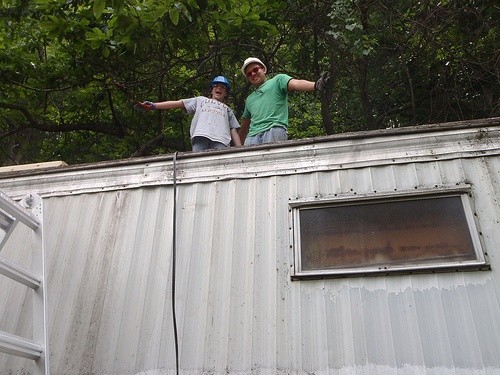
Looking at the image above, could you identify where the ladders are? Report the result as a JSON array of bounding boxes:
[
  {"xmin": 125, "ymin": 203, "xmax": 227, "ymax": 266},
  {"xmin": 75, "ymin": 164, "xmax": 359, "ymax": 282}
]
[{"xmin": 0, "ymin": 188, "xmax": 50, "ymax": 375}]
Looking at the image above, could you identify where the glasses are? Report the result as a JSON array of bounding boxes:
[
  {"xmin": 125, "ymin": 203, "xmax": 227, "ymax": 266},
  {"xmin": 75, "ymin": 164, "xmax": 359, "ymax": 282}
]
[
  {"xmin": 246, "ymin": 67, "xmax": 263, "ymax": 77},
  {"xmin": 212, "ymin": 85, "xmax": 227, "ymax": 89}
]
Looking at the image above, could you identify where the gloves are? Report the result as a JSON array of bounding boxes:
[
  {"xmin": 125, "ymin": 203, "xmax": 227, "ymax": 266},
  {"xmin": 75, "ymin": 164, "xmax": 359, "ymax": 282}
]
[
  {"xmin": 314, "ymin": 71, "xmax": 331, "ymax": 94},
  {"xmin": 139, "ymin": 101, "xmax": 155, "ymax": 110}
]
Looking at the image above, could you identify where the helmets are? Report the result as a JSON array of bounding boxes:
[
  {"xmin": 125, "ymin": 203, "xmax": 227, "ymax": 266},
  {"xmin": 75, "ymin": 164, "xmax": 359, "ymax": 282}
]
[{"xmin": 210, "ymin": 76, "xmax": 230, "ymax": 92}]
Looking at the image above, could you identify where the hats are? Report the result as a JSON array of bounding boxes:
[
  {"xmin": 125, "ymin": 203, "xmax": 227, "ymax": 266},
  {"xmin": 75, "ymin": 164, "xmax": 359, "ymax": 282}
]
[{"xmin": 241, "ymin": 57, "xmax": 267, "ymax": 78}]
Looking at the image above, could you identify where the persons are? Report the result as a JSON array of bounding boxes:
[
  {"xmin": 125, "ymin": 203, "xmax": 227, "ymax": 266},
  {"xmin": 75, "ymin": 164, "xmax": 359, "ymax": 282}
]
[
  {"xmin": 138, "ymin": 76, "xmax": 242, "ymax": 152},
  {"xmin": 240, "ymin": 57, "xmax": 330, "ymax": 145}
]
